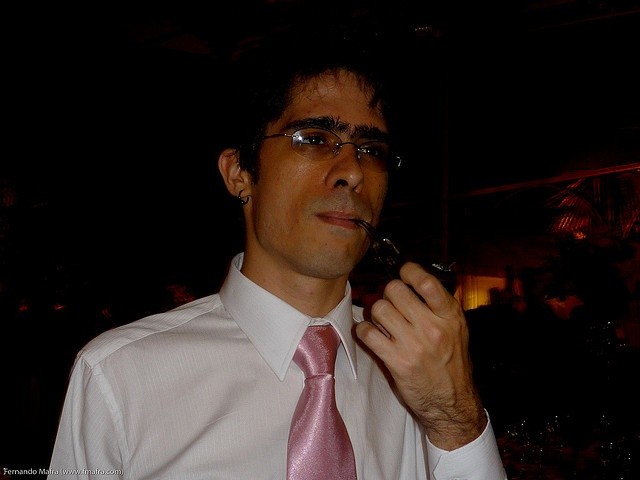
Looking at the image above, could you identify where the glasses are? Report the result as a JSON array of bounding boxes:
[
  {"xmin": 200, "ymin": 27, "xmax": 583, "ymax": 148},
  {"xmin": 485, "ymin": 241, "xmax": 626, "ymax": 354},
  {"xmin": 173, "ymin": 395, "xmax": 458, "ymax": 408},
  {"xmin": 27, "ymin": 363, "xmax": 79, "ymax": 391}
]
[{"xmin": 261, "ymin": 126, "xmax": 393, "ymax": 169}]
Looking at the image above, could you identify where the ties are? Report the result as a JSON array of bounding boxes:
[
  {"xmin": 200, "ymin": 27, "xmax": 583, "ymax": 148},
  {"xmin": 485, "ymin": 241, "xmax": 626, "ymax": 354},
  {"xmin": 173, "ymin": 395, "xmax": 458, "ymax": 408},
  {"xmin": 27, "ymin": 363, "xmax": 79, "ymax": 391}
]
[{"xmin": 288, "ymin": 327, "xmax": 357, "ymax": 480}]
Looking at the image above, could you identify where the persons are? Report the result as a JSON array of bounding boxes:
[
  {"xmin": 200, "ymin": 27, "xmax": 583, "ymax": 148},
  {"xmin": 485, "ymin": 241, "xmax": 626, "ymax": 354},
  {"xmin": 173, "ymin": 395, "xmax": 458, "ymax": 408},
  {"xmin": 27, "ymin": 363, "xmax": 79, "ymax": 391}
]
[{"xmin": 47, "ymin": 39, "xmax": 509, "ymax": 480}]
